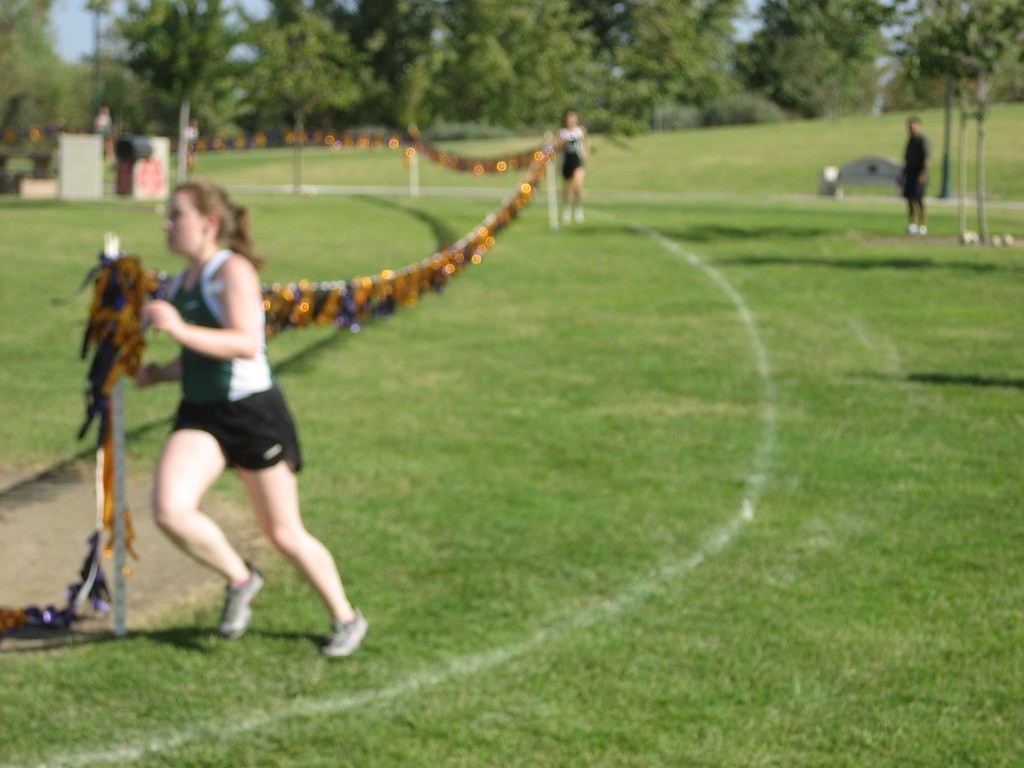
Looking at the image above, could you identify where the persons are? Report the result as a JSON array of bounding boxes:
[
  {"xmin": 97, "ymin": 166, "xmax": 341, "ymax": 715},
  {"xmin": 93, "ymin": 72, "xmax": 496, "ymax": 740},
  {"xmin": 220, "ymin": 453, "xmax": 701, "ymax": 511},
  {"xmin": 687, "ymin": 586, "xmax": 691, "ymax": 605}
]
[
  {"xmin": 186, "ymin": 119, "xmax": 201, "ymax": 171},
  {"xmin": 93, "ymin": 105, "xmax": 112, "ymax": 152},
  {"xmin": 138, "ymin": 178, "xmax": 365, "ymax": 657},
  {"xmin": 897, "ymin": 117, "xmax": 932, "ymax": 235},
  {"xmin": 556, "ymin": 110, "xmax": 591, "ymax": 226}
]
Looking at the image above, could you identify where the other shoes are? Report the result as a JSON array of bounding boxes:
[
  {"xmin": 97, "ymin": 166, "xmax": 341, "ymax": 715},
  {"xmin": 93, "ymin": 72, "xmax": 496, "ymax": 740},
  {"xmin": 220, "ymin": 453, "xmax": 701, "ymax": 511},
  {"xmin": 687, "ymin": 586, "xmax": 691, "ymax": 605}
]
[
  {"xmin": 908, "ymin": 223, "xmax": 927, "ymax": 235},
  {"xmin": 562, "ymin": 208, "xmax": 572, "ymax": 226},
  {"xmin": 574, "ymin": 206, "xmax": 584, "ymax": 223}
]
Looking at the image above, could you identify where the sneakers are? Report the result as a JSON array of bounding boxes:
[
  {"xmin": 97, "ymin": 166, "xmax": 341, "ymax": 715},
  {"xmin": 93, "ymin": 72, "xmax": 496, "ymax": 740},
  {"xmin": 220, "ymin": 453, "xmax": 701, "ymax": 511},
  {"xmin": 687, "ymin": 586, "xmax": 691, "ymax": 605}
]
[
  {"xmin": 219, "ymin": 561, "xmax": 265, "ymax": 640},
  {"xmin": 320, "ymin": 606, "xmax": 369, "ymax": 656}
]
